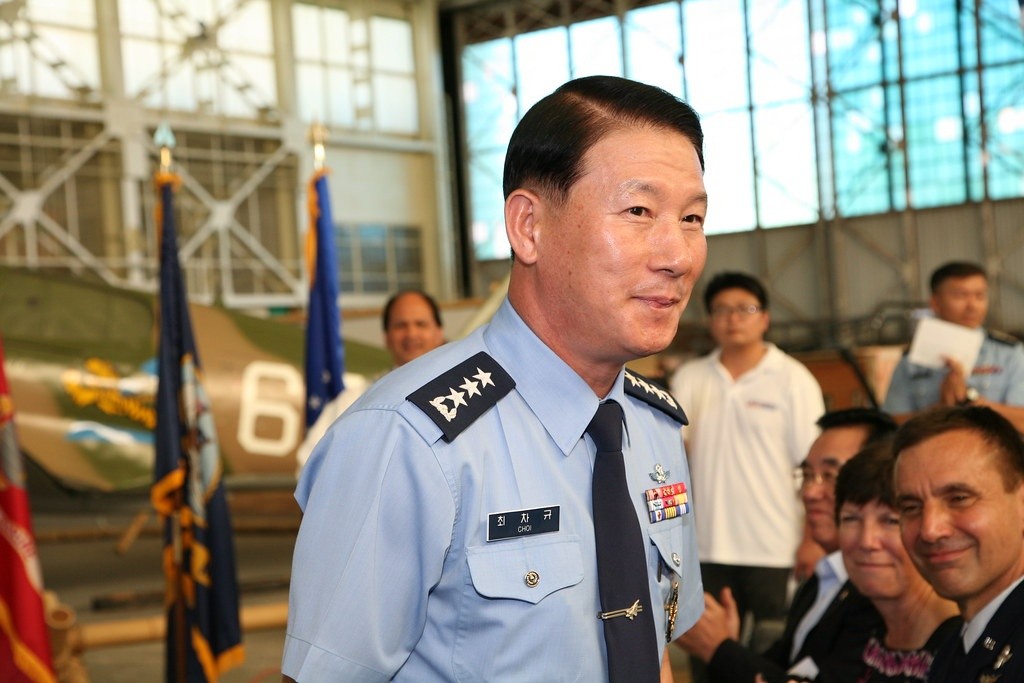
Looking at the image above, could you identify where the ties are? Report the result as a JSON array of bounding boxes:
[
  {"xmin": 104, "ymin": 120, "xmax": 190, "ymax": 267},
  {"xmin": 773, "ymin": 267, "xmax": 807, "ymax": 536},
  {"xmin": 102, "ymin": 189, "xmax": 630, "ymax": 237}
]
[{"xmin": 586, "ymin": 397, "xmax": 664, "ymax": 682}]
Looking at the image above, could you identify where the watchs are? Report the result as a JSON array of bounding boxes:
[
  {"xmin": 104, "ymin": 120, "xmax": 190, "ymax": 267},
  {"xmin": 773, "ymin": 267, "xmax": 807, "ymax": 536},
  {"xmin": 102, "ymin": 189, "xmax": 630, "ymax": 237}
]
[{"xmin": 958, "ymin": 385, "xmax": 981, "ymax": 407}]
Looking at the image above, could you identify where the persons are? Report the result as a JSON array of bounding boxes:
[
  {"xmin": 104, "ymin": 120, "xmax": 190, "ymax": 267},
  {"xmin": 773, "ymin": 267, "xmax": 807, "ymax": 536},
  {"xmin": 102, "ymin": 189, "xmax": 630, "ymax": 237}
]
[
  {"xmin": 279, "ymin": 76, "xmax": 710, "ymax": 683},
  {"xmin": 833, "ymin": 442, "xmax": 965, "ymax": 683},
  {"xmin": 883, "ymin": 401, "xmax": 1024, "ymax": 682},
  {"xmin": 674, "ymin": 402, "xmax": 887, "ymax": 683},
  {"xmin": 665, "ymin": 267, "xmax": 830, "ymax": 683},
  {"xmin": 878, "ymin": 258, "xmax": 1024, "ymax": 436},
  {"xmin": 379, "ymin": 285, "xmax": 446, "ymax": 379}
]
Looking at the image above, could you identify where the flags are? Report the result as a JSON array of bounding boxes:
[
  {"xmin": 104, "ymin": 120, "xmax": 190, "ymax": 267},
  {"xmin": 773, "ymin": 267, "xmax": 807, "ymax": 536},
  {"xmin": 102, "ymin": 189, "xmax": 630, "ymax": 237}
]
[
  {"xmin": 148, "ymin": 145, "xmax": 247, "ymax": 682},
  {"xmin": 295, "ymin": 122, "xmax": 345, "ymax": 445},
  {"xmin": 0, "ymin": 346, "xmax": 62, "ymax": 682}
]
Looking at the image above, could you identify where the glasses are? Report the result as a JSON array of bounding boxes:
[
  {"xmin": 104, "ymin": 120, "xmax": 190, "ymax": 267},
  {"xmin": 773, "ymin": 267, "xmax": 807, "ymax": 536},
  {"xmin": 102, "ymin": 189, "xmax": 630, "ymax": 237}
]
[
  {"xmin": 711, "ymin": 302, "xmax": 762, "ymax": 319},
  {"xmin": 792, "ymin": 465, "xmax": 838, "ymax": 495}
]
